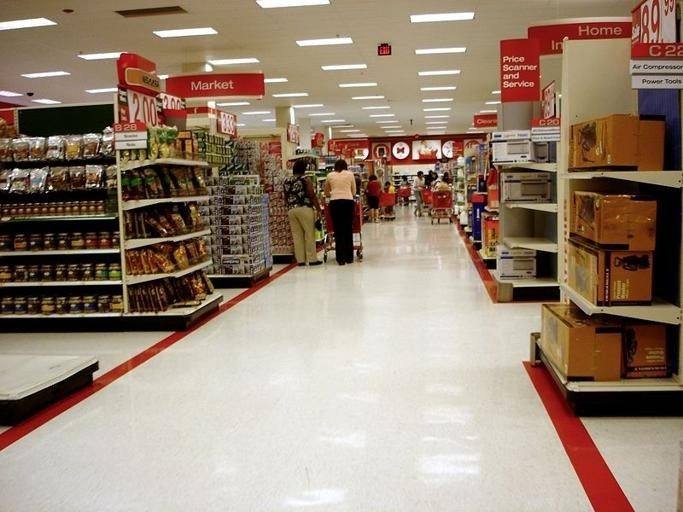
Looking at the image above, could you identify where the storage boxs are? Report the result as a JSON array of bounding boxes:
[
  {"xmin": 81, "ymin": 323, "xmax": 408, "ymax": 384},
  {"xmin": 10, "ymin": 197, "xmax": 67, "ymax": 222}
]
[{"xmin": 538, "ymin": 112, "xmax": 667, "ymax": 402}]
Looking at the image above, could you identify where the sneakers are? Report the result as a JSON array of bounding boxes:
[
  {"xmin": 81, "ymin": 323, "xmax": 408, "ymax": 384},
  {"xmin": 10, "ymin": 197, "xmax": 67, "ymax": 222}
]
[{"xmin": 298, "ymin": 262, "xmax": 322, "ymax": 266}]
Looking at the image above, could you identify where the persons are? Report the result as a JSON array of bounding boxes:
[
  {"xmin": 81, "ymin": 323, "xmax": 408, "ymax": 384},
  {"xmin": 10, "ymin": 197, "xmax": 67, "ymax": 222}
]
[
  {"xmin": 354, "ymin": 170, "xmax": 449, "ymax": 223},
  {"xmin": 324, "ymin": 160, "xmax": 357, "ymax": 265},
  {"xmin": 283, "ymin": 160, "xmax": 323, "ymax": 266}
]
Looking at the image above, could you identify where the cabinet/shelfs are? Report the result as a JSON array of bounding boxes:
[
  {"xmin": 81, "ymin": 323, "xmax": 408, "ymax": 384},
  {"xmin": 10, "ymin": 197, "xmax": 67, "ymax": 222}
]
[
  {"xmin": 122, "ymin": 152, "xmax": 223, "ymax": 327},
  {"xmin": 488, "ymin": 157, "xmax": 559, "ymax": 294},
  {"xmin": 1, "ymin": 211, "xmax": 121, "ymax": 318}
]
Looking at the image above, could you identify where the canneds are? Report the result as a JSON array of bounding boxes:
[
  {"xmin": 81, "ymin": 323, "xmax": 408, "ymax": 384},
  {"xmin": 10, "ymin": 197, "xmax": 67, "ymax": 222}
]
[
  {"xmin": 0, "ymin": 294, "xmax": 123, "ymax": 315},
  {"xmin": 0, "ymin": 231, "xmax": 120, "ymax": 252},
  {"xmin": 0, "ymin": 262, "xmax": 121, "ymax": 282}
]
